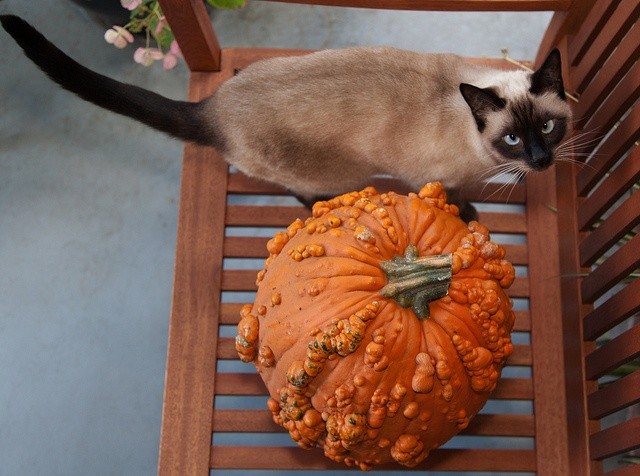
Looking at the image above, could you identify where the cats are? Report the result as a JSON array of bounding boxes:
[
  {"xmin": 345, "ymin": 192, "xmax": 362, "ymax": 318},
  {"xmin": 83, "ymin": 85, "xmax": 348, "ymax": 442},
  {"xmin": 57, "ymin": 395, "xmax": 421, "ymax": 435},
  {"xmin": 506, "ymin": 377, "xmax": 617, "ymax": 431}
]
[{"xmin": 0, "ymin": 12, "xmax": 608, "ymax": 223}]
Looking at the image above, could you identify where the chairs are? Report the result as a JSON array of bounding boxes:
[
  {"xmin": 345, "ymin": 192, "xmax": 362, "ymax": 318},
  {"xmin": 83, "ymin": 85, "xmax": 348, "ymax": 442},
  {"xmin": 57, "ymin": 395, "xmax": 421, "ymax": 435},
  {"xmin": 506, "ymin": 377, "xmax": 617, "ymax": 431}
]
[{"xmin": 157, "ymin": 0, "xmax": 640, "ymax": 476}]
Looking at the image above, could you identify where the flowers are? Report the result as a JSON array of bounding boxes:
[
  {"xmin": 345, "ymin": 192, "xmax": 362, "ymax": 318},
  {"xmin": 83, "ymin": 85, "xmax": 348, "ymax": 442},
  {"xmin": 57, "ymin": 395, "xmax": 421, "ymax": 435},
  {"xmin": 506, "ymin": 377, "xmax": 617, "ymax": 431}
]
[{"xmin": 103, "ymin": 0, "xmax": 247, "ymax": 70}]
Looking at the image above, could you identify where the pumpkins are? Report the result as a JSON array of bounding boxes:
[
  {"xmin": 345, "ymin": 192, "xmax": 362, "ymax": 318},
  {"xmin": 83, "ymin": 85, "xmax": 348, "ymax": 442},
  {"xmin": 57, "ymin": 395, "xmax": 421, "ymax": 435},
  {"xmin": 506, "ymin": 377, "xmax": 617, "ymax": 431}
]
[{"xmin": 234, "ymin": 181, "xmax": 516, "ymax": 472}]
